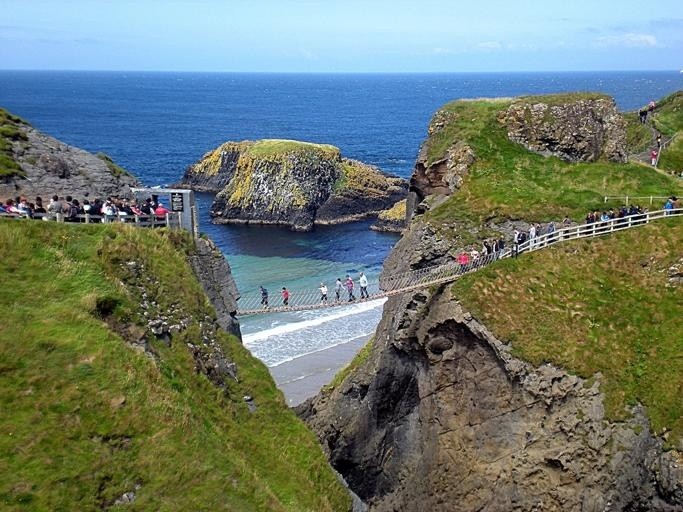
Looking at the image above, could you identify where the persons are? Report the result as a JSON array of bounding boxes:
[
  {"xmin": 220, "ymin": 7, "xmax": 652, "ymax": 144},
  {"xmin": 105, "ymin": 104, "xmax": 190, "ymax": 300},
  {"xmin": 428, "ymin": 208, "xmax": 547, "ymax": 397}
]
[
  {"xmin": 344, "ymin": 275, "xmax": 357, "ymax": 303},
  {"xmin": 280, "ymin": 286, "xmax": 288, "ymax": 309},
  {"xmin": 455, "ymin": 205, "xmax": 647, "ymax": 273},
  {"xmin": 639, "ymin": 100, "xmax": 655, "ymax": 123},
  {"xmin": 655, "ymin": 130, "xmax": 661, "ymax": 148},
  {"xmin": 651, "ymin": 148, "xmax": 658, "ymax": 166},
  {"xmin": 665, "ymin": 196, "xmax": 681, "ymax": 216},
  {"xmin": 259, "ymin": 286, "xmax": 269, "ymax": 310},
  {"xmin": 318, "ymin": 282, "xmax": 328, "ymax": 306},
  {"xmin": 335, "ymin": 278, "xmax": 346, "ymax": 302},
  {"xmin": 0, "ymin": 195, "xmax": 176, "ymax": 222},
  {"xmin": 356, "ymin": 272, "xmax": 369, "ymax": 300}
]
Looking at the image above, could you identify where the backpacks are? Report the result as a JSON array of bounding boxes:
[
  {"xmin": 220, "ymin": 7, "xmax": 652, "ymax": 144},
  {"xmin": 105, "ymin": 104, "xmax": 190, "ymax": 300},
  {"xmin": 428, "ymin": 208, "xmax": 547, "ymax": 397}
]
[
  {"xmin": 667, "ymin": 204, "xmax": 672, "ymax": 209},
  {"xmin": 67, "ymin": 202, "xmax": 77, "ymax": 217}
]
